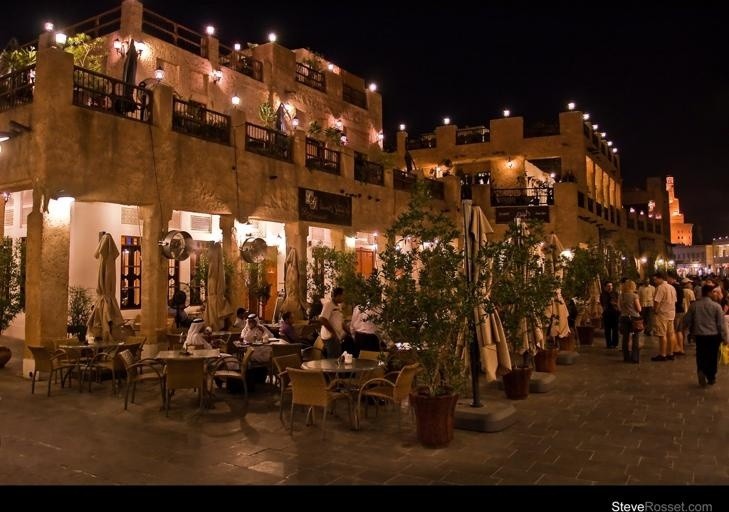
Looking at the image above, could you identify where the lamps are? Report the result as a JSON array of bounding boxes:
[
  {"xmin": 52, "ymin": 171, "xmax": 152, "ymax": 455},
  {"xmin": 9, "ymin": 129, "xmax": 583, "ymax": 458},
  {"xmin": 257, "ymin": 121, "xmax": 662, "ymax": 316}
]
[
  {"xmin": 137, "ymin": 40, "xmax": 147, "ymax": 56},
  {"xmin": 113, "ymin": 37, "xmax": 122, "ymax": 53},
  {"xmin": 212, "ymin": 66, "xmax": 221, "ymax": 86},
  {"xmin": 153, "ymin": 64, "xmax": 165, "ymax": 83}
]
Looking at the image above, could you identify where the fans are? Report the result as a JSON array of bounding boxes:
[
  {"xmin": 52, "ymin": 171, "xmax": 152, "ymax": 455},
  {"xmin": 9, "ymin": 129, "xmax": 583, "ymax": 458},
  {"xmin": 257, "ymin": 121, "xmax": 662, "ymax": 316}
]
[
  {"xmin": 158, "ymin": 229, "xmax": 193, "ymax": 261},
  {"xmin": 238, "ymin": 237, "xmax": 267, "ymax": 264}
]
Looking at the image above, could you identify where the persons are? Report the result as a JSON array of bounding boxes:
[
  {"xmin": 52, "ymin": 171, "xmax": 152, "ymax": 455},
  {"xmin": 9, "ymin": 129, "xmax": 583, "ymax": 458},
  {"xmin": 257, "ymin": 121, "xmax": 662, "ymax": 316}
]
[
  {"xmin": 599, "ymin": 271, "xmax": 729, "ymax": 387},
  {"xmin": 168, "ymin": 286, "xmax": 385, "ymax": 395}
]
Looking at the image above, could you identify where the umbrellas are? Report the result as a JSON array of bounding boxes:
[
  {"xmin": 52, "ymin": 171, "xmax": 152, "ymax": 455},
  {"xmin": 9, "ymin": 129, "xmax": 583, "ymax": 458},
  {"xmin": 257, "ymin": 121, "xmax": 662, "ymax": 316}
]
[
  {"xmin": 114, "ymin": 38, "xmax": 139, "ymax": 114},
  {"xmin": 279, "ymin": 248, "xmax": 310, "ymax": 321},
  {"xmin": 539, "ymin": 231, "xmax": 571, "ymax": 339},
  {"xmin": 504, "ymin": 219, "xmax": 545, "ymax": 356},
  {"xmin": 570, "ymin": 242, "xmax": 623, "ymax": 327},
  {"xmin": 86, "ymin": 233, "xmax": 124, "ymax": 344},
  {"xmin": 202, "ymin": 241, "xmax": 234, "ymax": 330},
  {"xmin": 454, "ymin": 206, "xmax": 512, "ymax": 383}
]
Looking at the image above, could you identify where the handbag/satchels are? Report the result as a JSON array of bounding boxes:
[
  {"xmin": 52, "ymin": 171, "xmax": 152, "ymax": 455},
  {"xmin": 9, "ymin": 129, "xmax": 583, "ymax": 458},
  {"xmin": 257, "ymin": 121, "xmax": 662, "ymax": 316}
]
[{"xmin": 626, "ymin": 318, "xmax": 644, "ymax": 332}]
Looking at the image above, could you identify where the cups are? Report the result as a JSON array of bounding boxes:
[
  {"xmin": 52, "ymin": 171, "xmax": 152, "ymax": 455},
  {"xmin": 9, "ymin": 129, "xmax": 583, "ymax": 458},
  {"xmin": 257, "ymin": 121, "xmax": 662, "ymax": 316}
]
[{"xmin": 344, "ymin": 353, "xmax": 352, "ymax": 364}]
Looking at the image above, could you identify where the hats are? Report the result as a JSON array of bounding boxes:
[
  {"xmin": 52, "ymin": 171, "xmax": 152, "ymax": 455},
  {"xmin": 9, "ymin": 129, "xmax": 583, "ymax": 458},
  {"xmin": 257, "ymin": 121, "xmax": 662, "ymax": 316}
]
[
  {"xmin": 701, "ymin": 283, "xmax": 717, "ymax": 296},
  {"xmin": 681, "ymin": 277, "xmax": 694, "ymax": 284}
]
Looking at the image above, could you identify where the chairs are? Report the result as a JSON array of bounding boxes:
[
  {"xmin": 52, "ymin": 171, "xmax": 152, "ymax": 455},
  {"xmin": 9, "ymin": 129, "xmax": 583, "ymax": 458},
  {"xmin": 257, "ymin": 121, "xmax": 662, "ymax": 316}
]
[{"xmin": 25, "ymin": 316, "xmax": 421, "ymax": 439}]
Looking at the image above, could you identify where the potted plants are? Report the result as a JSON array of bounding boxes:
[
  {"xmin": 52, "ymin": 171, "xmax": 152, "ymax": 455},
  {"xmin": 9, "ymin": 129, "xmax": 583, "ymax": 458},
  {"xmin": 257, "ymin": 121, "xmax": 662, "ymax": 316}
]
[{"xmin": 361, "ymin": 180, "xmax": 603, "ymax": 450}]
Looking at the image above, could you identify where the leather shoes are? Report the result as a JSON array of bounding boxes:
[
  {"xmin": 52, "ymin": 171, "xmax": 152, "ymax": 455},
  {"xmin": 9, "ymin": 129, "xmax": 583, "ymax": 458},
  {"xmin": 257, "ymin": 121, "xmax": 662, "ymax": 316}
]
[{"xmin": 609, "ymin": 346, "xmax": 616, "ymax": 348}]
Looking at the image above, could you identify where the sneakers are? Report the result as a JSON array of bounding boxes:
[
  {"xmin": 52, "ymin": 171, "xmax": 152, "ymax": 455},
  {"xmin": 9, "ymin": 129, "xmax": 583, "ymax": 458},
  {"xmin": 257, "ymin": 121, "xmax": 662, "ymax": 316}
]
[
  {"xmin": 643, "ymin": 328, "xmax": 691, "ymax": 361},
  {"xmin": 708, "ymin": 379, "xmax": 715, "ymax": 385},
  {"xmin": 696, "ymin": 371, "xmax": 706, "ymax": 386}
]
